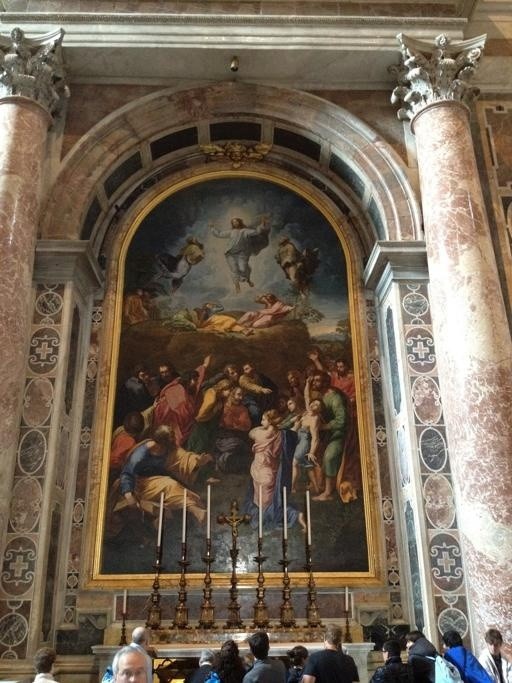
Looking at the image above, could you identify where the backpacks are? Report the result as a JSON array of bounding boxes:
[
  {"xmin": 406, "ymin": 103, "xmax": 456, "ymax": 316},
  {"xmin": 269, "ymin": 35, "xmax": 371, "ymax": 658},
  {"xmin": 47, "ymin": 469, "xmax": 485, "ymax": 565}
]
[{"xmin": 425, "ymin": 653, "xmax": 465, "ymax": 683}]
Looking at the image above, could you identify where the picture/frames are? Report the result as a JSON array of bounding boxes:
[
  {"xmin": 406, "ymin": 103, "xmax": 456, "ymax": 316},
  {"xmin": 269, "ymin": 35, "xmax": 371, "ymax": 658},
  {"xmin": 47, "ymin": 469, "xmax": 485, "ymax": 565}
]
[{"xmin": 84, "ymin": 152, "xmax": 387, "ymax": 582}]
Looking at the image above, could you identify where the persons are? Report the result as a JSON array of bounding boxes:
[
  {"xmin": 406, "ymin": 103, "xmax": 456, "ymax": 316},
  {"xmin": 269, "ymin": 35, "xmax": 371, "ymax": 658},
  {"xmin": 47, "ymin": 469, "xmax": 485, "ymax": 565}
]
[{"xmin": 31, "ymin": 622, "xmax": 512, "ymax": 683}]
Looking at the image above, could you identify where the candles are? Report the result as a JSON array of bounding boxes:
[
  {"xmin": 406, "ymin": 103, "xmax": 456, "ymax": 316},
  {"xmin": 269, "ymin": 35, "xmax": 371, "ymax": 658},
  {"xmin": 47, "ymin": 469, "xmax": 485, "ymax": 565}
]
[{"xmin": 122, "ymin": 484, "xmax": 349, "ymax": 614}]
